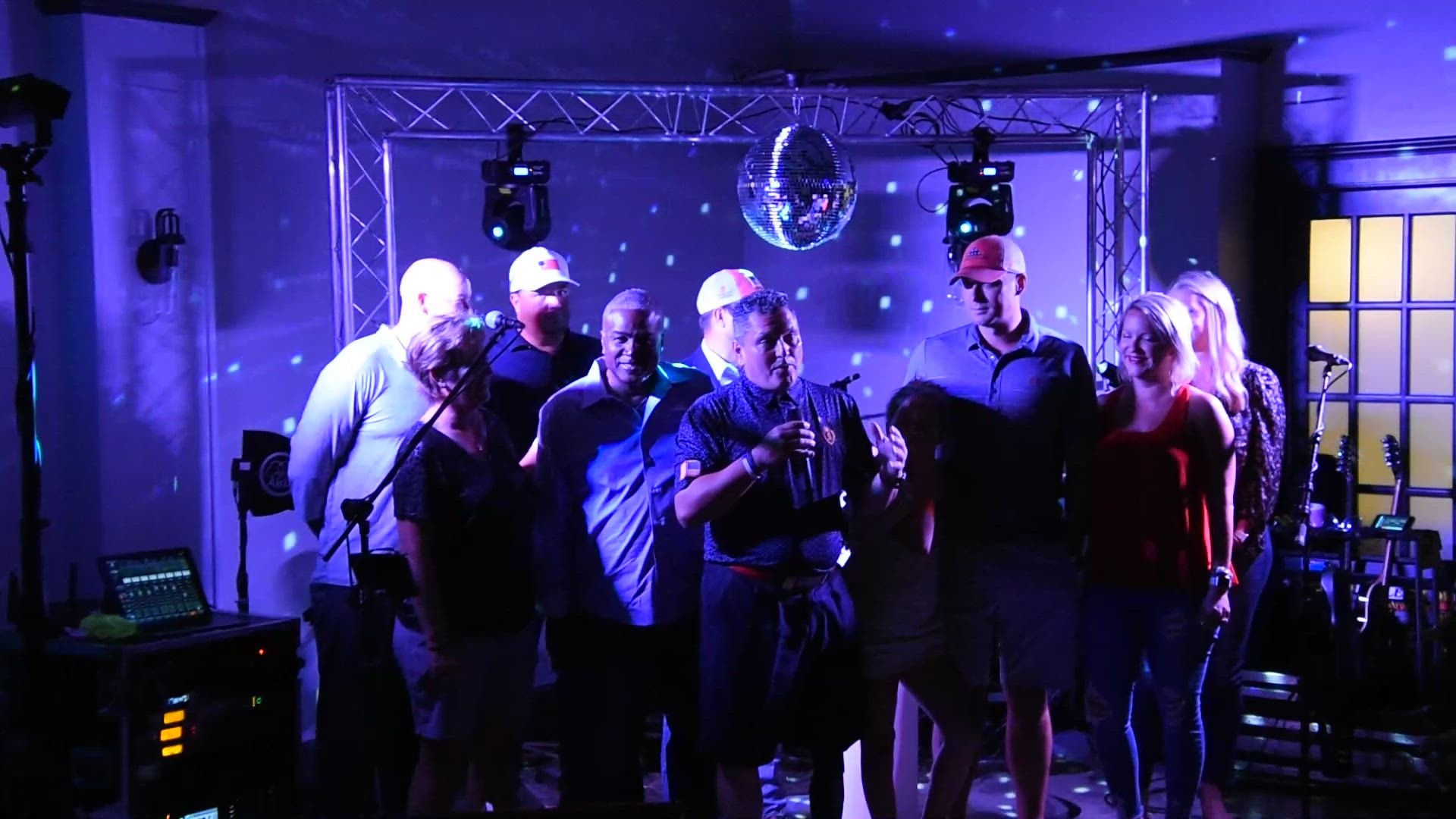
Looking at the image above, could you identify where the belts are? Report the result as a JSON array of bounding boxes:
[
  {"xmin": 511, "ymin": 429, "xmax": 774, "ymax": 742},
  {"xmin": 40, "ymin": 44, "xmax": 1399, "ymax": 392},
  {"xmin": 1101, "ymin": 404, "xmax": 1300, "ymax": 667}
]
[{"xmin": 728, "ymin": 562, "xmax": 837, "ymax": 592}]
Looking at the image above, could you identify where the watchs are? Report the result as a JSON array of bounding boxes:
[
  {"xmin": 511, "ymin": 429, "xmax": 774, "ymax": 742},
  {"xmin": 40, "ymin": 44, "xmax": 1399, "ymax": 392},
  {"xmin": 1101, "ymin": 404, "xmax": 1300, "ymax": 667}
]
[{"xmin": 1209, "ymin": 575, "xmax": 1232, "ymax": 589}]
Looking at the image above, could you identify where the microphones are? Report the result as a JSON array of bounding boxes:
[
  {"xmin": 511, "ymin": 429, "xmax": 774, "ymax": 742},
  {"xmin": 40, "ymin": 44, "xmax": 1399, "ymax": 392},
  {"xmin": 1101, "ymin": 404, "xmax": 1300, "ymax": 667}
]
[
  {"xmin": 1305, "ymin": 344, "xmax": 1349, "ymax": 366},
  {"xmin": 829, "ymin": 373, "xmax": 862, "ymax": 389},
  {"xmin": 485, "ymin": 309, "xmax": 526, "ymax": 331}
]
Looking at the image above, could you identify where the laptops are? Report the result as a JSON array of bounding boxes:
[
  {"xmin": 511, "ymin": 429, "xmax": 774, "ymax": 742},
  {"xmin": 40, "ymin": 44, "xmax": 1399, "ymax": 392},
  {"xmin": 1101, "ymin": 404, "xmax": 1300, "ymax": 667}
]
[{"xmin": 97, "ymin": 547, "xmax": 249, "ymax": 640}]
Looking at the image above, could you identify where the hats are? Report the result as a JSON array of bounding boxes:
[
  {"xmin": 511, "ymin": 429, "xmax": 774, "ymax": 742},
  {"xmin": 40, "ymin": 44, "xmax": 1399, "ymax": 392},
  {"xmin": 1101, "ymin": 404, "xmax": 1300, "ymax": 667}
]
[
  {"xmin": 695, "ymin": 268, "xmax": 764, "ymax": 315},
  {"xmin": 508, "ymin": 246, "xmax": 581, "ymax": 292},
  {"xmin": 948, "ymin": 235, "xmax": 1026, "ymax": 287}
]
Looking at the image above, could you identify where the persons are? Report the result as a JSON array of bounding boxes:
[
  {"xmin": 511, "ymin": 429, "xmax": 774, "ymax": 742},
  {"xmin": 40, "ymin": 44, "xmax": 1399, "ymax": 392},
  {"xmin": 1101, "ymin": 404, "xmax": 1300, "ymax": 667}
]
[
  {"xmin": 640, "ymin": 269, "xmax": 766, "ymax": 819},
  {"xmin": 808, "ymin": 377, "xmax": 982, "ymax": 819},
  {"xmin": 531, "ymin": 289, "xmax": 691, "ymax": 819},
  {"xmin": 482, "ymin": 244, "xmax": 604, "ymax": 714},
  {"xmin": 287, "ymin": 256, "xmax": 474, "ymax": 819},
  {"xmin": 904, "ymin": 235, "xmax": 1097, "ymax": 819},
  {"xmin": 1093, "ymin": 293, "xmax": 1239, "ymax": 819},
  {"xmin": 674, "ymin": 290, "xmax": 908, "ymax": 819},
  {"xmin": 391, "ymin": 315, "xmax": 529, "ymax": 819},
  {"xmin": 1133, "ymin": 271, "xmax": 1287, "ymax": 819}
]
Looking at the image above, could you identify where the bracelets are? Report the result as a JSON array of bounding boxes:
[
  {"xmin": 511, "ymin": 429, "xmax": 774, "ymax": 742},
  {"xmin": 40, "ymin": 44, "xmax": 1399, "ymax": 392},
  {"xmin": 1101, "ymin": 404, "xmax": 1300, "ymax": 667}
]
[
  {"xmin": 427, "ymin": 642, "xmax": 441, "ymax": 652},
  {"xmin": 1215, "ymin": 566, "xmax": 1233, "ymax": 577},
  {"xmin": 1234, "ymin": 529, "xmax": 1248, "ymax": 545},
  {"xmin": 741, "ymin": 451, "xmax": 760, "ymax": 480},
  {"xmin": 878, "ymin": 460, "xmax": 907, "ymax": 488}
]
